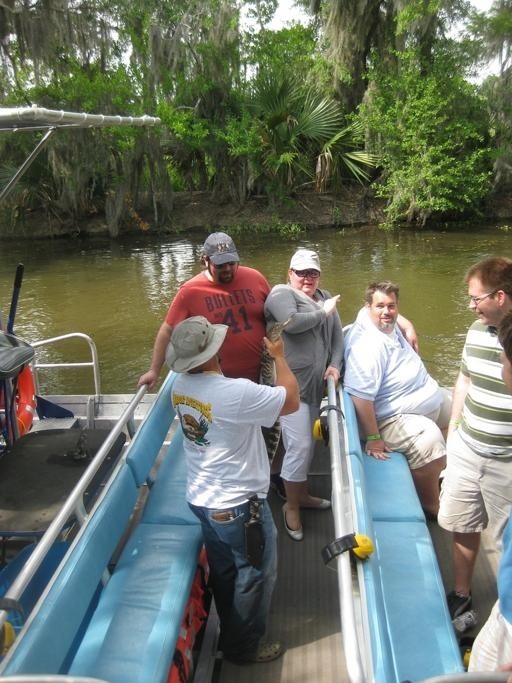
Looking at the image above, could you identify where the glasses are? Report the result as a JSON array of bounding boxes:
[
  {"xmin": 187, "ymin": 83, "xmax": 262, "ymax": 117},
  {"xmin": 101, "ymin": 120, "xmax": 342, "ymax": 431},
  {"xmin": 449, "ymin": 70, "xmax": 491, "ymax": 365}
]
[
  {"xmin": 472, "ymin": 289, "xmax": 500, "ymax": 305},
  {"xmin": 291, "ymin": 269, "xmax": 320, "ymax": 278}
]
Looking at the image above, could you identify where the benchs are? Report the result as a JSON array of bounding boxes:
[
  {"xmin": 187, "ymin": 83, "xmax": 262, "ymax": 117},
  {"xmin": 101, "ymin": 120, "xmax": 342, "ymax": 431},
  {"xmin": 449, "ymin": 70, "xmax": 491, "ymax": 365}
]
[
  {"xmin": 327, "ymin": 408, "xmax": 467, "ymax": 683},
  {"xmin": 124, "ymin": 352, "xmax": 209, "ymax": 525},
  {"xmin": 0, "ymin": 415, "xmax": 210, "ymax": 683},
  {"xmin": 326, "ymin": 372, "xmax": 428, "ymax": 520}
]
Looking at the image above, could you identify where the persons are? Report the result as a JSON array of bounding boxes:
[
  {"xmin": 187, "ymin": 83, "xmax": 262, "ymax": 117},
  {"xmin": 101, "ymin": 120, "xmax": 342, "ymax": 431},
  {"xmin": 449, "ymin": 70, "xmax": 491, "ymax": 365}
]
[
  {"xmin": 437, "ymin": 258, "xmax": 511, "ymax": 623},
  {"xmin": 466, "ymin": 307, "xmax": 512, "ymax": 683},
  {"xmin": 264, "ymin": 249, "xmax": 345, "ymax": 541},
  {"xmin": 137, "ymin": 230, "xmax": 286, "ymax": 502},
  {"xmin": 344, "ymin": 279, "xmax": 453, "ymax": 520},
  {"xmin": 164, "ymin": 314, "xmax": 300, "ymax": 665}
]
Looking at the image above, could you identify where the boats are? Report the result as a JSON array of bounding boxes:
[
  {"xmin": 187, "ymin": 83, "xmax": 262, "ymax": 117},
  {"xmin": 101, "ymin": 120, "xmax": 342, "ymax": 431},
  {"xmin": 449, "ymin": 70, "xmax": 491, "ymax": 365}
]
[{"xmin": 2, "ymin": 104, "xmax": 512, "ymax": 683}]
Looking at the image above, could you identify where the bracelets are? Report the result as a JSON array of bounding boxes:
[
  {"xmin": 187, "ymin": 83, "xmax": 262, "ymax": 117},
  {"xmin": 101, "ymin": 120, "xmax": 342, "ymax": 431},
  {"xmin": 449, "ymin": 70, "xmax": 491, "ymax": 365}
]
[
  {"xmin": 450, "ymin": 419, "xmax": 460, "ymax": 426},
  {"xmin": 366, "ymin": 432, "xmax": 380, "ymax": 441}
]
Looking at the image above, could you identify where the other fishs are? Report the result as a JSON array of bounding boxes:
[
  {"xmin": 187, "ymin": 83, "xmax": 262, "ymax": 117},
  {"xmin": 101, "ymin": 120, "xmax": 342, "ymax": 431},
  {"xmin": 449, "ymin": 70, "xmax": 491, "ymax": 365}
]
[{"xmin": 259, "ymin": 313, "xmax": 293, "ymax": 467}]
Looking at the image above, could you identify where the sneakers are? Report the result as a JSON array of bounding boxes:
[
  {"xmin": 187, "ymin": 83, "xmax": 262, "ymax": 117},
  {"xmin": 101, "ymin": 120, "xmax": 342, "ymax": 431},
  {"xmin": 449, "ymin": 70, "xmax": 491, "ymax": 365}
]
[
  {"xmin": 446, "ymin": 590, "xmax": 473, "ymax": 620},
  {"xmin": 245, "ymin": 639, "xmax": 282, "ymax": 662}
]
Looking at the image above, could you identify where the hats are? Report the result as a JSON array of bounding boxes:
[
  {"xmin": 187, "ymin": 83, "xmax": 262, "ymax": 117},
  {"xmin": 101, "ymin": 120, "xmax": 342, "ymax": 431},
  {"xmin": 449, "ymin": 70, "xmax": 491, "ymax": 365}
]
[
  {"xmin": 165, "ymin": 315, "xmax": 228, "ymax": 373},
  {"xmin": 289, "ymin": 249, "xmax": 322, "ymax": 271},
  {"xmin": 204, "ymin": 232, "xmax": 240, "ymax": 265}
]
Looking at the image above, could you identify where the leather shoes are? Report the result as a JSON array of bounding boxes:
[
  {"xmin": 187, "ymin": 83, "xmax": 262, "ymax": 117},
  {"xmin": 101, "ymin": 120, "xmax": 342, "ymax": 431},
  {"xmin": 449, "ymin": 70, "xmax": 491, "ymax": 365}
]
[{"xmin": 282, "ymin": 499, "xmax": 331, "ymax": 541}]
